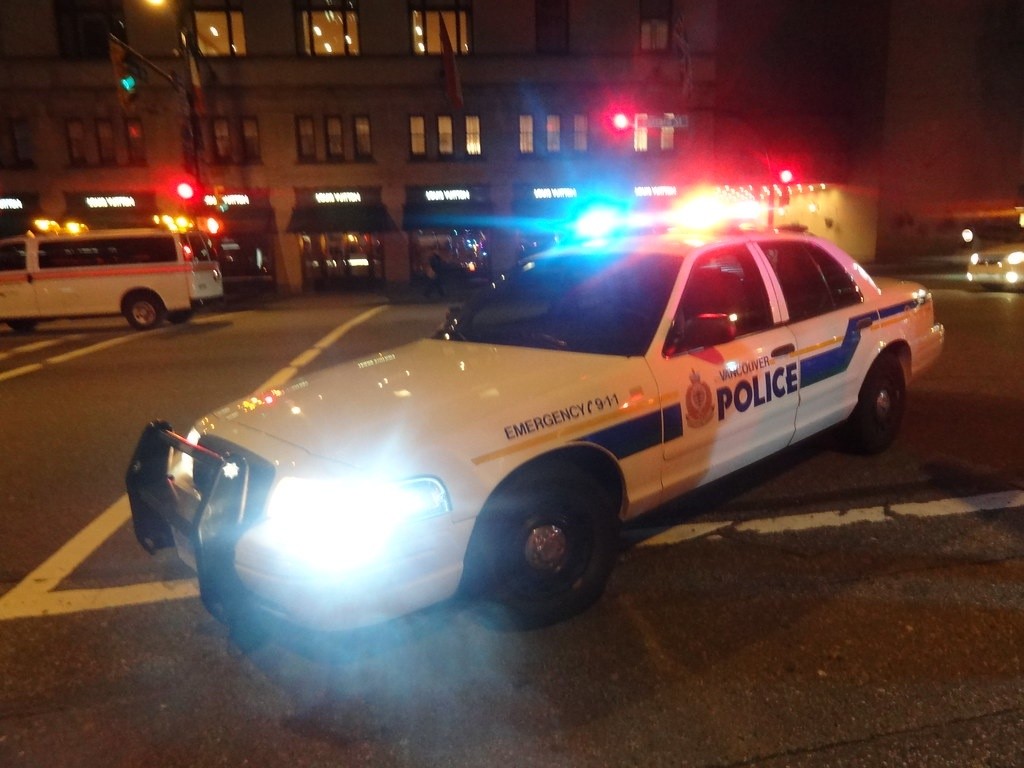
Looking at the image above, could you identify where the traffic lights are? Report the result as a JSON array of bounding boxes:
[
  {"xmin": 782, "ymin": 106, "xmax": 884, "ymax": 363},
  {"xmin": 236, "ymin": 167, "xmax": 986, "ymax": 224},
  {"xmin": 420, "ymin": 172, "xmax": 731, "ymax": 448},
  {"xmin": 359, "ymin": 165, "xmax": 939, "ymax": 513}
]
[
  {"xmin": 176, "ymin": 181, "xmax": 198, "ymax": 203},
  {"xmin": 107, "ymin": 41, "xmax": 139, "ymax": 116},
  {"xmin": 604, "ymin": 105, "xmax": 637, "ymax": 136}
]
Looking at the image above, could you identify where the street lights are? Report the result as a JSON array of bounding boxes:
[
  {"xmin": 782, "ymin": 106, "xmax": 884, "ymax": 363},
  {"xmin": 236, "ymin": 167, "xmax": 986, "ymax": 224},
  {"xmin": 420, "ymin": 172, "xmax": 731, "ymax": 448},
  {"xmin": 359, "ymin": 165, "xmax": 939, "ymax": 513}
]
[{"xmin": 766, "ymin": 169, "xmax": 793, "ymax": 231}]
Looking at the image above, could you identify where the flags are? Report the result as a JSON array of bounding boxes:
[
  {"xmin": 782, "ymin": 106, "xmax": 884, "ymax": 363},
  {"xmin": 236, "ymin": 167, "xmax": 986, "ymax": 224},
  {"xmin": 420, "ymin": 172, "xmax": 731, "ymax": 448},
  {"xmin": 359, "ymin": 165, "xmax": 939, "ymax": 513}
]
[
  {"xmin": 439, "ymin": 12, "xmax": 465, "ymax": 114},
  {"xmin": 184, "ymin": 38, "xmax": 205, "ymax": 151},
  {"xmin": 111, "ymin": 42, "xmax": 135, "ymax": 115}
]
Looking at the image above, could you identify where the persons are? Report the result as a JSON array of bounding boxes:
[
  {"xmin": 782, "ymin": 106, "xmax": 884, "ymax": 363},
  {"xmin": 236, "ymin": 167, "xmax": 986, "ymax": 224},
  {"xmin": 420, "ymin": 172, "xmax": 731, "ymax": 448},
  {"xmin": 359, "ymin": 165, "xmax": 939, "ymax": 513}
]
[{"xmin": 421, "ymin": 245, "xmax": 444, "ymax": 296}]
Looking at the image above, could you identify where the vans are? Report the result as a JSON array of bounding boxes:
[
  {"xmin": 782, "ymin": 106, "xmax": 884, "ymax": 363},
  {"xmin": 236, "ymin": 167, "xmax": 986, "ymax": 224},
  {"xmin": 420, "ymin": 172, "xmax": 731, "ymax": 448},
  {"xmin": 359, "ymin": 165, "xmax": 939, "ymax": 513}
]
[{"xmin": 0, "ymin": 228, "xmax": 226, "ymax": 331}]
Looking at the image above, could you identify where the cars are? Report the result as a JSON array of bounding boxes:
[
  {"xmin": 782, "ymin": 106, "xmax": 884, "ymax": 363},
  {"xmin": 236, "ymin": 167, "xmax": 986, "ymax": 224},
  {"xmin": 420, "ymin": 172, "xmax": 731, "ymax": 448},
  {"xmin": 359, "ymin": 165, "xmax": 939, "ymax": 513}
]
[
  {"xmin": 966, "ymin": 243, "xmax": 1024, "ymax": 292},
  {"xmin": 127, "ymin": 225, "xmax": 943, "ymax": 640}
]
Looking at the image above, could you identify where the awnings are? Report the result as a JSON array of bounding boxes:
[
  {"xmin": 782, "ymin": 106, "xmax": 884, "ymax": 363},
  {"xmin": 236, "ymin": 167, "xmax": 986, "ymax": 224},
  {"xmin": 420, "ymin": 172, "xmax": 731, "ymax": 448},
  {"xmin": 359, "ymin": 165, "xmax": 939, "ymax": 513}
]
[{"xmin": 174, "ymin": 203, "xmax": 497, "ymax": 234}]
